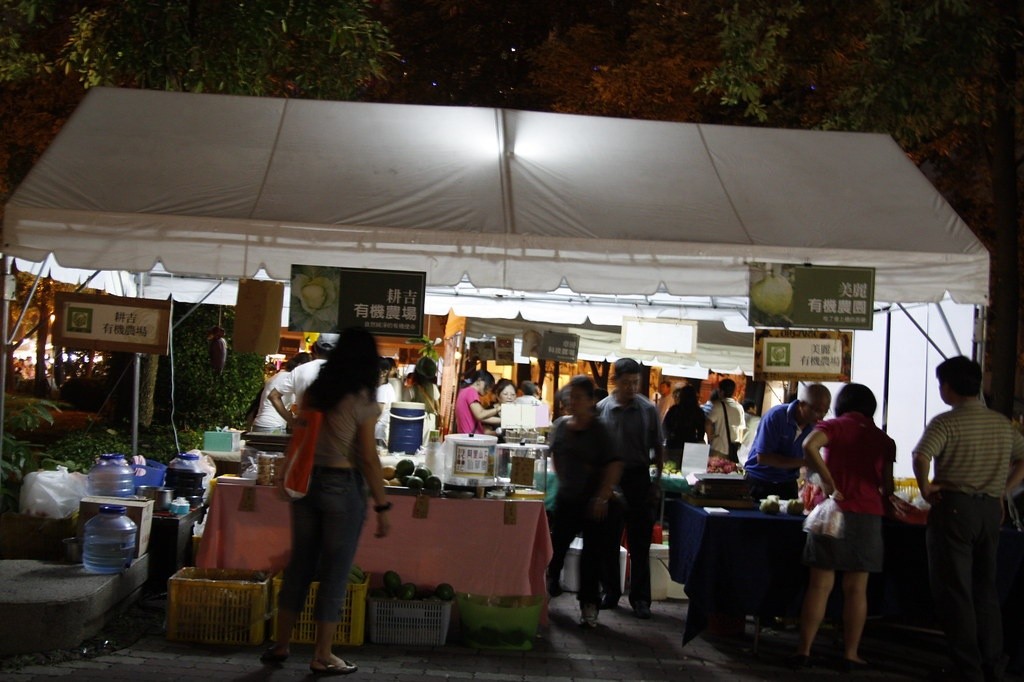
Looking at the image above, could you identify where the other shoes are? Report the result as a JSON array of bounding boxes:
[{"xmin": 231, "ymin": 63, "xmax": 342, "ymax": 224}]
[
  {"xmin": 632, "ymin": 598, "xmax": 650, "ymax": 620},
  {"xmin": 601, "ymin": 592, "xmax": 622, "ymax": 609},
  {"xmin": 548, "ymin": 580, "xmax": 561, "ymax": 597},
  {"xmin": 940, "ymin": 678, "xmax": 960, "ymax": 682},
  {"xmin": 580, "ymin": 603, "xmax": 599, "ymax": 626}
]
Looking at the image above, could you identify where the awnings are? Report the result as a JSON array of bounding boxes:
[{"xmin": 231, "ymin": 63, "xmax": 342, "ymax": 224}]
[{"xmin": 0, "ymin": 86, "xmax": 991, "ymax": 485}]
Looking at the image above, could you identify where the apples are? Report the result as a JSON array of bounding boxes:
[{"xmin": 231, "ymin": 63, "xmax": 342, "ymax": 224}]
[{"xmin": 382, "ymin": 466, "xmax": 402, "ymax": 486}]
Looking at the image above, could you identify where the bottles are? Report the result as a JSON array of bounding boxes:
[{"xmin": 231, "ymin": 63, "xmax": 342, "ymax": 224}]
[
  {"xmin": 88, "ymin": 452, "xmax": 133, "ymax": 496},
  {"xmin": 82, "ymin": 504, "xmax": 138, "ymax": 575},
  {"xmin": 164, "ymin": 454, "xmax": 206, "ymax": 507}
]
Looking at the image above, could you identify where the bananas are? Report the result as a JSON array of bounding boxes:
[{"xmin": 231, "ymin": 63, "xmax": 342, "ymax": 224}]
[
  {"xmin": 651, "ymin": 461, "xmax": 682, "ymax": 475},
  {"xmin": 346, "ymin": 565, "xmax": 366, "ymax": 586}
]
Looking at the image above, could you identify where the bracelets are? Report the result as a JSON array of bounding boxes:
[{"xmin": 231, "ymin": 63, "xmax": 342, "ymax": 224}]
[
  {"xmin": 374, "ymin": 501, "xmax": 393, "ymax": 512},
  {"xmin": 594, "ymin": 495, "xmax": 609, "ymax": 505}
]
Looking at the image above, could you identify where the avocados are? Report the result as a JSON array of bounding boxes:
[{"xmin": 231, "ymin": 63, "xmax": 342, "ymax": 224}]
[
  {"xmin": 397, "ymin": 459, "xmax": 442, "ymax": 490},
  {"xmin": 371, "ymin": 569, "xmax": 455, "ymax": 602}
]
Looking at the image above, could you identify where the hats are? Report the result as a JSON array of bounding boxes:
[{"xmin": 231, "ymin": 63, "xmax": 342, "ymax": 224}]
[{"xmin": 317, "ymin": 333, "xmax": 341, "ymax": 351}]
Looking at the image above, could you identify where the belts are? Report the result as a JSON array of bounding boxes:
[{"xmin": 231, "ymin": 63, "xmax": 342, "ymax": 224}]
[{"xmin": 948, "ymin": 489, "xmax": 983, "ymax": 499}]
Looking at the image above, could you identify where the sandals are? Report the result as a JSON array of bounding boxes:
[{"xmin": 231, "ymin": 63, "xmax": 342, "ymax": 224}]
[
  {"xmin": 310, "ymin": 656, "xmax": 358, "ymax": 676},
  {"xmin": 259, "ymin": 647, "xmax": 289, "ymax": 665}
]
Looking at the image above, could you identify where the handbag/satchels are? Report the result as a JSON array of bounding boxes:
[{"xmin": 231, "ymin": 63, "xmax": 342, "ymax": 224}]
[
  {"xmin": 802, "ymin": 492, "xmax": 845, "ymax": 539},
  {"xmin": 728, "ymin": 442, "xmax": 741, "ymax": 463},
  {"xmin": 275, "ymin": 387, "xmax": 323, "ymax": 498}
]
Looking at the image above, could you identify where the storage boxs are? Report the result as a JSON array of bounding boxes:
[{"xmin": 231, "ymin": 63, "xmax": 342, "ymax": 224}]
[
  {"xmin": 371, "ymin": 594, "xmax": 453, "ymax": 646},
  {"xmin": 650, "ymin": 545, "xmax": 689, "ymax": 602},
  {"xmin": 269, "ymin": 566, "xmax": 370, "ymax": 646},
  {"xmin": 167, "ymin": 566, "xmax": 279, "ymax": 646},
  {"xmin": 77, "ymin": 495, "xmax": 157, "ymax": 557}
]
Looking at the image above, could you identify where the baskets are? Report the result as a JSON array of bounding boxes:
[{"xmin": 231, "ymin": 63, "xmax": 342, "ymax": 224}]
[
  {"xmin": 367, "ymin": 592, "xmax": 455, "ymax": 646},
  {"xmin": 165, "ymin": 565, "xmax": 269, "ymax": 646},
  {"xmin": 270, "ymin": 572, "xmax": 370, "ymax": 646}
]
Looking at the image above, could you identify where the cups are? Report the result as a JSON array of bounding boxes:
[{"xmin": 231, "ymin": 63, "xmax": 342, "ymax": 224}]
[{"xmin": 427, "ymin": 430, "xmax": 441, "ymax": 477}]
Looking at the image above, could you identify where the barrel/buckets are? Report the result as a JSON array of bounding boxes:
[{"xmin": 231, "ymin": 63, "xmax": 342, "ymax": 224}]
[{"xmin": 388, "ymin": 401, "xmax": 426, "ymax": 454}]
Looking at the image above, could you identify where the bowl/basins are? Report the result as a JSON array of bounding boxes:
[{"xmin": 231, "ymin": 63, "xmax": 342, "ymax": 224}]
[{"xmin": 138, "ymin": 485, "xmax": 175, "ymax": 512}]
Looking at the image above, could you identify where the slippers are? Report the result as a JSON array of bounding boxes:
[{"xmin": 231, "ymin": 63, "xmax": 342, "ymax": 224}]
[
  {"xmin": 786, "ymin": 653, "xmax": 809, "ymax": 668},
  {"xmin": 842, "ymin": 655, "xmax": 869, "ymax": 674}
]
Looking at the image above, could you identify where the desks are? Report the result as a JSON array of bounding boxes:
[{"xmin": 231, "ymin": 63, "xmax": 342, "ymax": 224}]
[
  {"xmin": 533, "ymin": 466, "xmax": 696, "ymax": 529},
  {"xmin": 668, "ymin": 501, "xmax": 1023, "ymax": 654},
  {"xmin": 207, "ymin": 474, "xmax": 556, "ymax": 626}
]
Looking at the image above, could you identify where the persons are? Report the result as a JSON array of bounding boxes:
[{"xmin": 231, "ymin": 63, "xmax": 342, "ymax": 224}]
[
  {"xmin": 742, "ymin": 384, "xmax": 831, "ymax": 637},
  {"xmin": 789, "ymin": 384, "xmax": 896, "ymax": 667},
  {"xmin": 705, "ymin": 379, "xmax": 746, "ymax": 462},
  {"xmin": 656, "ymin": 381, "xmax": 744, "ymax": 471},
  {"xmin": 592, "ymin": 357, "xmax": 662, "ymax": 618},
  {"xmin": 662, "ymin": 385, "xmax": 705, "ymax": 463},
  {"xmin": 455, "ymin": 370, "xmax": 505, "ymax": 436},
  {"xmin": 912, "ymin": 356, "xmax": 1024, "ymax": 682},
  {"xmin": 737, "ymin": 400, "xmax": 764, "ymax": 467},
  {"xmin": 267, "ymin": 332, "xmax": 341, "ymax": 427},
  {"xmin": 657, "ymin": 382, "xmax": 676, "ymax": 416},
  {"xmin": 11, "ymin": 352, "xmax": 50, "ymax": 379},
  {"xmin": 261, "ymin": 329, "xmax": 394, "ymax": 674},
  {"xmin": 547, "ymin": 376, "xmax": 621, "ymax": 628},
  {"xmin": 250, "ymin": 350, "xmax": 607, "ymax": 447}
]
